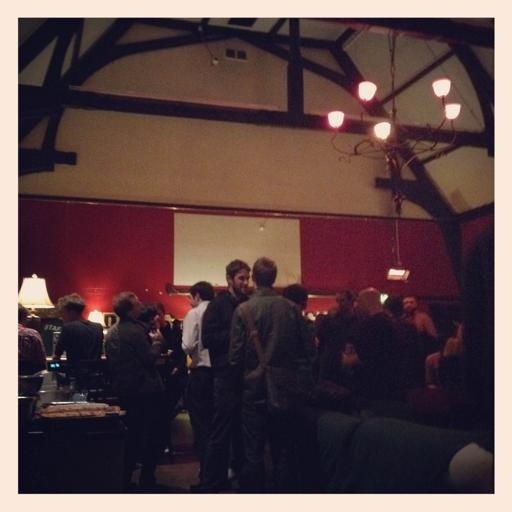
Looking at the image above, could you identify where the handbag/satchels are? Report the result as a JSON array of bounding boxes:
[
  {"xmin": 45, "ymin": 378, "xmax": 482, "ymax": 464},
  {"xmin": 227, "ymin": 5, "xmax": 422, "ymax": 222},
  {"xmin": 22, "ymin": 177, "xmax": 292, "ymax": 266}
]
[{"xmin": 262, "ymin": 362, "xmax": 316, "ymax": 416}]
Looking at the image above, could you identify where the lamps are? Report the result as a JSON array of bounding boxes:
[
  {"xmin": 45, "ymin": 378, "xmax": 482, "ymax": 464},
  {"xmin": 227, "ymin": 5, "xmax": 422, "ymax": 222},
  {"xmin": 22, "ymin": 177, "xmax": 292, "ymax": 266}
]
[
  {"xmin": 326, "ymin": 29, "xmax": 463, "ymax": 213},
  {"xmin": 18, "ymin": 273, "xmax": 56, "ymax": 319}
]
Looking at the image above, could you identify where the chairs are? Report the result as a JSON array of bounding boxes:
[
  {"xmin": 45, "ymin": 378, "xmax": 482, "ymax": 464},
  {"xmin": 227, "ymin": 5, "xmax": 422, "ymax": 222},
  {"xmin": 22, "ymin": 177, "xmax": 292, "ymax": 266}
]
[
  {"xmin": 19, "ymin": 361, "xmax": 152, "ymax": 493},
  {"xmin": 307, "ymin": 400, "xmax": 494, "ymax": 494}
]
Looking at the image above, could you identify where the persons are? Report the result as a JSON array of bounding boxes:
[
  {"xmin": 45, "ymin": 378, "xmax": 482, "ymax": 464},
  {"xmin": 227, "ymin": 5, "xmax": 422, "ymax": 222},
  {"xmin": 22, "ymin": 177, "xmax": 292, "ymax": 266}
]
[{"xmin": 19, "ymin": 257, "xmax": 494, "ymax": 493}]
